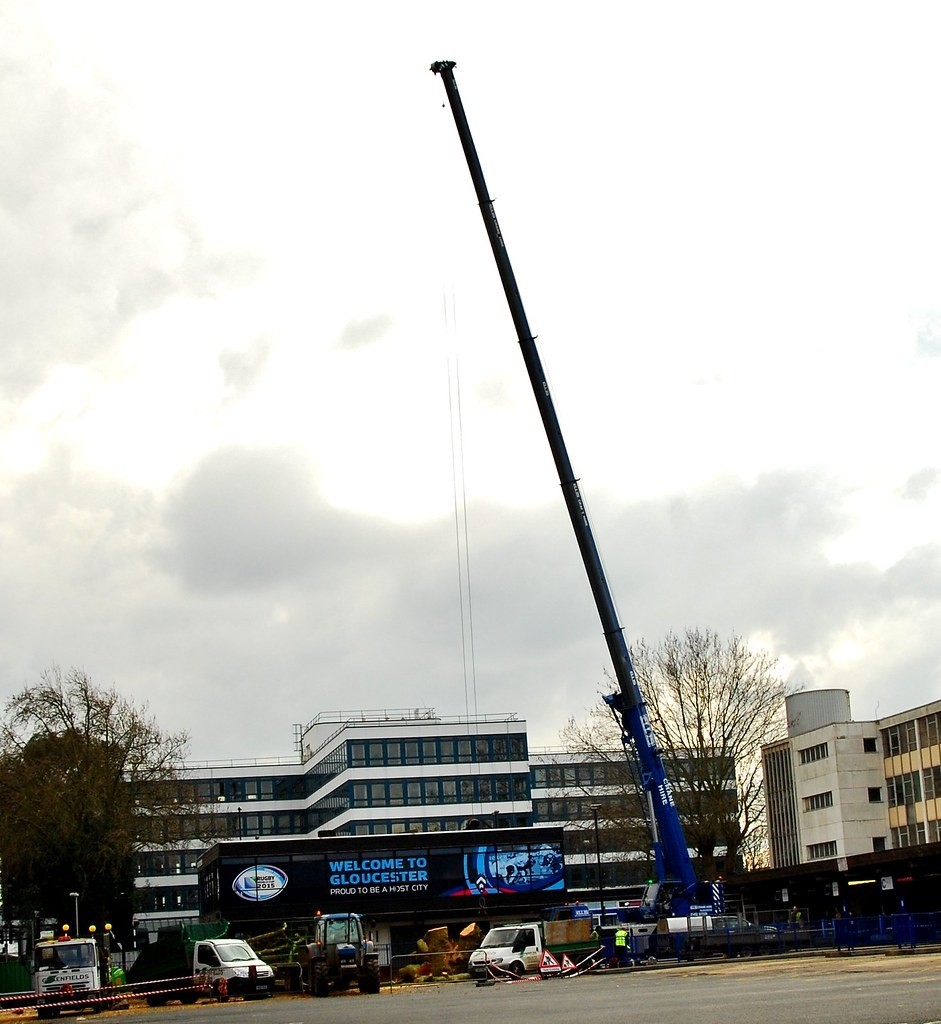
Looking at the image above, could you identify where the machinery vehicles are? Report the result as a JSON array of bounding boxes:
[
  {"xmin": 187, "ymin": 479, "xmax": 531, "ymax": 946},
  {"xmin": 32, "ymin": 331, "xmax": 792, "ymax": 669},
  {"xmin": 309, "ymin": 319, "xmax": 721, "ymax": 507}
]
[
  {"xmin": 306, "ymin": 911, "xmax": 380, "ymax": 998},
  {"xmin": 431, "ymin": 58, "xmax": 788, "ymax": 977},
  {"xmin": 131, "ymin": 937, "xmax": 275, "ymax": 1008},
  {"xmin": 35, "ymin": 938, "xmax": 111, "ymax": 1019}
]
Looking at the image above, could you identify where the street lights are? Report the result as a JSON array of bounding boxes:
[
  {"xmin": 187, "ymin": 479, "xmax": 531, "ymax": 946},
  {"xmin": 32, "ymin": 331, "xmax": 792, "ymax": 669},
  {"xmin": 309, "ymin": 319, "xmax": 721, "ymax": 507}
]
[
  {"xmin": 590, "ymin": 803, "xmax": 606, "ymax": 924},
  {"xmin": 69, "ymin": 892, "xmax": 79, "ymax": 938}
]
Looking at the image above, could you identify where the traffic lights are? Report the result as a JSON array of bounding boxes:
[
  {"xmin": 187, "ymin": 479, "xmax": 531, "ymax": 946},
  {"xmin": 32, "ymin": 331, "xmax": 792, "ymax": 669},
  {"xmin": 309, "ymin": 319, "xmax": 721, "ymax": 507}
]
[
  {"xmin": 717, "ymin": 874, "xmax": 724, "ymax": 882},
  {"xmin": 647, "ymin": 875, "xmax": 657, "ymax": 884}
]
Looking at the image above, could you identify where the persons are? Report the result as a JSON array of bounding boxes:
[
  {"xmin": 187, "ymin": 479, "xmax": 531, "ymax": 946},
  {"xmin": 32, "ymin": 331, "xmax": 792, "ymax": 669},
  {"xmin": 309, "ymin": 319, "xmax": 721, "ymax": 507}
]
[
  {"xmin": 614, "ymin": 924, "xmax": 629, "ymax": 966},
  {"xmin": 517, "ymin": 931, "xmax": 534, "ymax": 949},
  {"xmin": 848, "ymin": 911, "xmax": 854, "ymax": 919},
  {"xmin": 655, "ymin": 888, "xmax": 671, "ymax": 913},
  {"xmin": 788, "ymin": 906, "xmax": 804, "ymax": 930},
  {"xmin": 596, "ymin": 926, "xmax": 612, "ymax": 938}
]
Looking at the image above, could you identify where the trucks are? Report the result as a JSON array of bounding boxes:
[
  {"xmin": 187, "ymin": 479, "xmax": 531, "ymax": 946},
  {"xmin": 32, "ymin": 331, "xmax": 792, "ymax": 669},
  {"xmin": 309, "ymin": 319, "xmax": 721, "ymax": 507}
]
[{"xmin": 468, "ymin": 917, "xmax": 603, "ymax": 980}]
